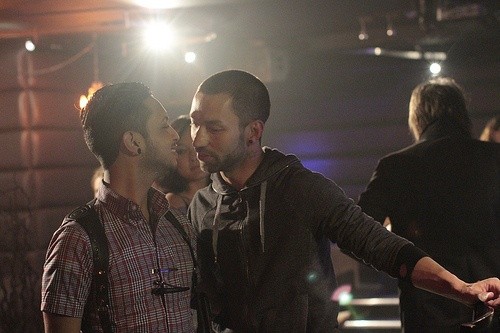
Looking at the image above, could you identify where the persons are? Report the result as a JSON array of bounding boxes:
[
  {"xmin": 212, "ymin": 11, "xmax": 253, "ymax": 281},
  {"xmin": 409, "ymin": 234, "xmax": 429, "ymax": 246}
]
[
  {"xmin": 153, "ymin": 114, "xmax": 209, "ymax": 218},
  {"xmin": 41, "ymin": 83, "xmax": 202, "ymax": 333},
  {"xmin": 94, "ymin": 167, "xmax": 105, "ymax": 198},
  {"xmin": 357, "ymin": 79, "xmax": 500, "ymax": 333},
  {"xmin": 187, "ymin": 70, "xmax": 500, "ymax": 333}
]
